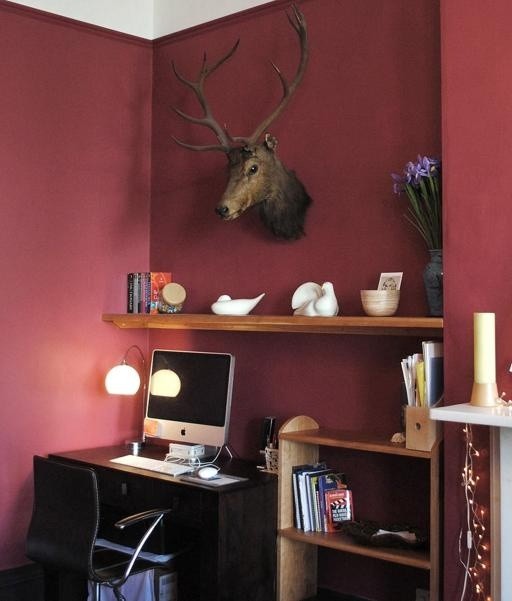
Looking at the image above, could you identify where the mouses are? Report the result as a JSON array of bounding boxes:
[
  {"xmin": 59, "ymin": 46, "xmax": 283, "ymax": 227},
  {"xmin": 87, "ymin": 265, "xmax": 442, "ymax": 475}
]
[{"xmin": 196, "ymin": 467, "xmax": 218, "ymax": 480}]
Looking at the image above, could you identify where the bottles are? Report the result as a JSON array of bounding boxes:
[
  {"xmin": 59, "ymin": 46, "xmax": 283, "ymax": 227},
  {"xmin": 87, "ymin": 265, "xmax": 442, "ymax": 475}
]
[{"xmin": 158, "ymin": 283, "xmax": 186, "ymax": 313}]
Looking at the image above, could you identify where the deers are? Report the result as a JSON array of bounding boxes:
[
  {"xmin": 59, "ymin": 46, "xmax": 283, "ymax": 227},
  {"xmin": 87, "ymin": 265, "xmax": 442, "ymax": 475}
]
[{"xmin": 166, "ymin": 0, "xmax": 314, "ymax": 242}]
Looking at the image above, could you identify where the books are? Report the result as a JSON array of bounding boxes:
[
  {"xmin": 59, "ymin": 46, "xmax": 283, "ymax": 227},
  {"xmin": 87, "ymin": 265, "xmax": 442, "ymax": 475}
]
[
  {"xmin": 291, "ymin": 462, "xmax": 354, "ymax": 535},
  {"xmin": 128, "ymin": 273, "xmax": 172, "ymax": 315}
]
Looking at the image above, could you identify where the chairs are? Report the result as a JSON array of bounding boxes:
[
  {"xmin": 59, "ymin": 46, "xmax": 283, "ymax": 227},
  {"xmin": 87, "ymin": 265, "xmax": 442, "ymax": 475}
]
[{"xmin": 28, "ymin": 455, "xmax": 179, "ymax": 601}]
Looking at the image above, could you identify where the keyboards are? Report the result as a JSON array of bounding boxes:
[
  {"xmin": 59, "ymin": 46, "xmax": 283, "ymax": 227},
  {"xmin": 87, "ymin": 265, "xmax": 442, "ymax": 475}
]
[{"xmin": 108, "ymin": 454, "xmax": 191, "ymax": 478}]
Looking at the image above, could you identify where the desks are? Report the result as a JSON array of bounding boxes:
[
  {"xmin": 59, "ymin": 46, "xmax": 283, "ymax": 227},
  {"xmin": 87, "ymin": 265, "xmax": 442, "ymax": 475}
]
[{"xmin": 52, "ymin": 439, "xmax": 278, "ymax": 601}]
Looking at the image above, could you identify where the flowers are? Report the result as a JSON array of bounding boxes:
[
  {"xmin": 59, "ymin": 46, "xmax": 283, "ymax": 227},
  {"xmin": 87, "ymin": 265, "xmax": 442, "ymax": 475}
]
[{"xmin": 393, "ymin": 153, "xmax": 443, "ymax": 251}]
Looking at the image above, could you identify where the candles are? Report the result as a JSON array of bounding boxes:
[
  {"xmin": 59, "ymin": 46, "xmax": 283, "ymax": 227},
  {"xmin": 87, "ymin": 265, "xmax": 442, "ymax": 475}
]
[{"xmin": 473, "ymin": 310, "xmax": 496, "ymax": 385}]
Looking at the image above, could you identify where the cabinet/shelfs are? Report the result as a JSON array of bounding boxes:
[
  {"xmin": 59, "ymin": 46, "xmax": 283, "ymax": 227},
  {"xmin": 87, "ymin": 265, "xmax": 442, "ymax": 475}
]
[{"xmin": 273, "ymin": 415, "xmax": 442, "ymax": 601}]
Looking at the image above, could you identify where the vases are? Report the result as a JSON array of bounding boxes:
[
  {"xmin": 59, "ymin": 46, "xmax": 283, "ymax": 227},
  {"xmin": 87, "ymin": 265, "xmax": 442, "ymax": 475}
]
[{"xmin": 421, "ymin": 251, "xmax": 443, "ymax": 318}]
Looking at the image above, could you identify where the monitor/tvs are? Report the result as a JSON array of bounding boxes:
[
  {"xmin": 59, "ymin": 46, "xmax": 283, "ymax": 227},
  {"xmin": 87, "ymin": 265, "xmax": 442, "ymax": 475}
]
[{"xmin": 144, "ymin": 349, "xmax": 236, "ymax": 466}]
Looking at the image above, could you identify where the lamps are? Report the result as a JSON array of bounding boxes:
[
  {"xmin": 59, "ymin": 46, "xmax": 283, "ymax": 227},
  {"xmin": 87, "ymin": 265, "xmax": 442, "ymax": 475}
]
[
  {"xmin": 105, "ymin": 344, "xmax": 150, "ymax": 447},
  {"xmin": 150, "ymin": 354, "xmax": 182, "ymax": 398}
]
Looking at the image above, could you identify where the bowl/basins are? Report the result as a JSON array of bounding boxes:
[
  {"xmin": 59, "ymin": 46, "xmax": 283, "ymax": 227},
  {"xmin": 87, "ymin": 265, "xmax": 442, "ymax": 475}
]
[{"xmin": 360, "ymin": 288, "xmax": 401, "ymax": 317}]
